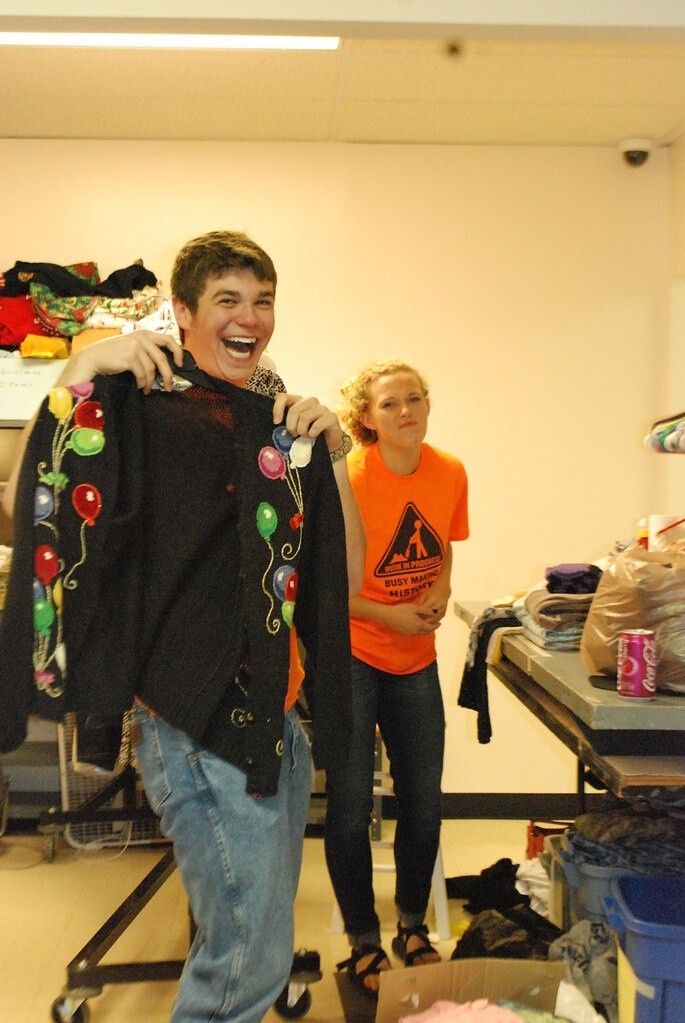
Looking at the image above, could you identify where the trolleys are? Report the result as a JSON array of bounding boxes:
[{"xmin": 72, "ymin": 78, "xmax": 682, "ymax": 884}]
[{"xmin": 39, "ymin": 724, "xmax": 327, "ymax": 1023}]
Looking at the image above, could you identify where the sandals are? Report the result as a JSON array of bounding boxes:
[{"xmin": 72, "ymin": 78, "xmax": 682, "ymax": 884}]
[
  {"xmin": 391, "ymin": 920, "xmax": 437, "ymax": 968},
  {"xmin": 335, "ymin": 945, "xmax": 387, "ymax": 1000}
]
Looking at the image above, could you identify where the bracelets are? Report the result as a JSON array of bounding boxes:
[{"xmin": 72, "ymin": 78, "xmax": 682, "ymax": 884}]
[{"xmin": 321, "ymin": 427, "xmax": 354, "ymax": 461}]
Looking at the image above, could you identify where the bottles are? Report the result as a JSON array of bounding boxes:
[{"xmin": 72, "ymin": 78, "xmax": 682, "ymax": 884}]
[{"xmin": 637, "ymin": 531, "xmax": 648, "ymax": 551}]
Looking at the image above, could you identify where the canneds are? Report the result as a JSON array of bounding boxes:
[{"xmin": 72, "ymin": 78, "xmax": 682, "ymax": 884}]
[{"xmin": 617, "ymin": 628, "xmax": 656, "ymax": 701}]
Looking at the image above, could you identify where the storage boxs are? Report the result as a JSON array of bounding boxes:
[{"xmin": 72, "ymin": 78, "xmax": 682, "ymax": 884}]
[
  {"xmin": 558, "ymin": 831, "xmax": 633, "ymax": 926},
  {"xmin": 0, "ymin": 327, "xmax": 126, "ymax": 421},
  {"xmin": 600, "ymin": 877, "xmax": 685, "ymax": 1023},
  {"xmin": 537, "ymin": 834, "xmax": 564, "ymax": 929},
  {"xmin": 376, "ymin": 956, "xmax": 610, "ymax": 1023}
]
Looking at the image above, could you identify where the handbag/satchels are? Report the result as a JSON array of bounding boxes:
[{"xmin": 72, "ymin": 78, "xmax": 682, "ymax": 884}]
[{"xmin": 579, "ymin": 539, "xmax": 684, "ymax": 692}]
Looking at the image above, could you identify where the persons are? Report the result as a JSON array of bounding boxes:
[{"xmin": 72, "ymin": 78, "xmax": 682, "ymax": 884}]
[
  {"xmin": 1, "ymin": 232, "xmax": 366, "ymax": 1023},
  {"xmin": 310, "ymin": 358, "xmax": 470, "ymax": 1000}
]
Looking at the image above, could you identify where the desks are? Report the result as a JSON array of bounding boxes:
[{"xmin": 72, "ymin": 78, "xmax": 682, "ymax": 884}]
[{"xmin": 454, "ymin": 600, "xmax": 685, "ymax": 817}]
